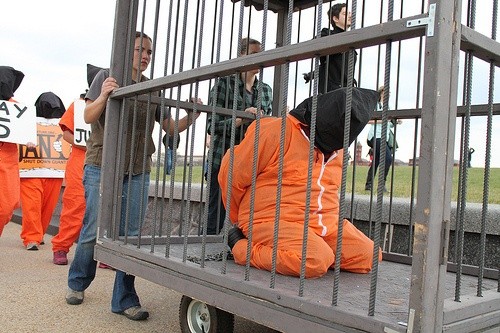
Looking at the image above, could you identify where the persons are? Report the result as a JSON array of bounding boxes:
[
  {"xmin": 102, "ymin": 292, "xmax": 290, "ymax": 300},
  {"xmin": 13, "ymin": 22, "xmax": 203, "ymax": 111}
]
[
  {"xmin": 162, "ymin": 133, "xmax": 180, "ymax": 176},
  {"xmin": 0, "ymin": 70, "xmax": 36, "ymax": 238},
  {"xmin": 21, "ymin": 94, "xmax": 64, "ymax": 250},
  {"xmin": 64, "ymin": 31, "xmax": 203, "ymax": 320},
  {"xmin": 364, "ymin": 85, "xmax": 402, "ymax": 193},
  {"xmin": 201, "ymin": 37, "xmax": 274, "ymax": 235},
  {"xmin": 51, "ymin": 68, "xmax": 111, "ymax": 269},
  {"xmin": 218, "ymin": 92, "xmax": 382, "ymax": 278},
  {"xmin": 204, "ymin": 134, "xmax": 211, "ymax": 183},
  {"xmin": 313, "ymin": 3, "xmax": 358, "ymax": 93}
]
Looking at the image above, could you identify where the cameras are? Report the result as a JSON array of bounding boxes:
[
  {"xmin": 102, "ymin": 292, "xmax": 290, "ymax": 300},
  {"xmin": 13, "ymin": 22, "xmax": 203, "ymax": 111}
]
[{"xmin": 302, "ymin": 71, "xmax": 314, "ymax": 83}]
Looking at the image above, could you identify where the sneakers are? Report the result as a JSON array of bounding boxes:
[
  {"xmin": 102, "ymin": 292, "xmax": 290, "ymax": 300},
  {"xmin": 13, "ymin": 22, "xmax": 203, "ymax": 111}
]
[
  {"xmin": 26, "ymin": 238, "xmax": 45, "ymax": 250},
  {"xmin": 66, "ymin": 284, "xmax": 84, "ymax": 305},
  {"xmin": 120, "ymin": 304, "xmax": 149, "ymax": 320},
  {"xmin": 53, "ymin": 250, "xmax": 68, "ymax": 265}
]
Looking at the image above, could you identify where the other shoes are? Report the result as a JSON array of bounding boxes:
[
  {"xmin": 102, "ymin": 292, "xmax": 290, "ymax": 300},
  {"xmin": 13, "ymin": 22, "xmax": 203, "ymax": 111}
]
[
  {"xmin": 223, "ymin": 227, "xmax": 245, "ymax": 249},
  {"xmin": 383, "ymin": 187, "xmax": 391, "ymax": 192},
  {"xmin": 365, "ymin": 184, "xmax": 372, "ymax": 191}
]
